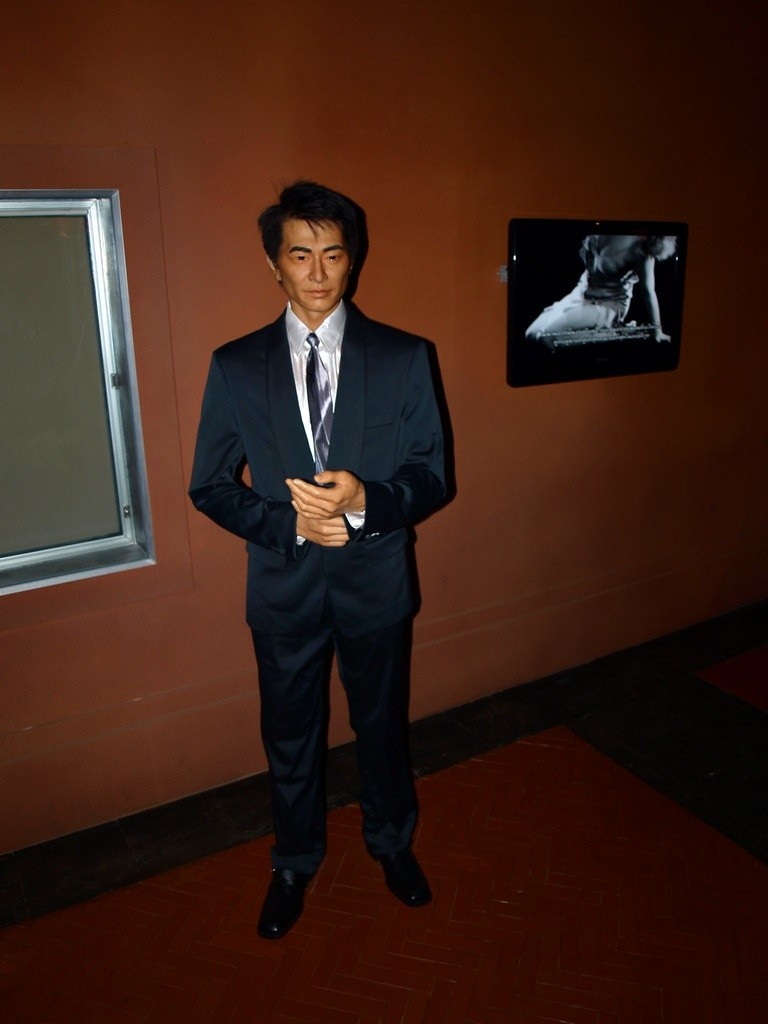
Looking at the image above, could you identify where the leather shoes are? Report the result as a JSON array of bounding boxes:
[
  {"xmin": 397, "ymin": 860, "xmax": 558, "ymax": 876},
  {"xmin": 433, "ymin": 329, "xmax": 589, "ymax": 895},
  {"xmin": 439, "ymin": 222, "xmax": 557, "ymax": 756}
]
[
  {"xmin": 377, "ymin": 848, "xmax": 433, "ymax": 907},
  {"xmin": 257, "ymin": 867, "xmax": 306, "ymax": 939}
]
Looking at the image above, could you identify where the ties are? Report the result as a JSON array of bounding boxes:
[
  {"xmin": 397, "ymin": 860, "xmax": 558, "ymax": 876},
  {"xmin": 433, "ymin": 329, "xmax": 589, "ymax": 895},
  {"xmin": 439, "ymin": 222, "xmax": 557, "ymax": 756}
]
[{"xmin": 307, "ymin": 333, "xmax": 335, "ymax": 474}]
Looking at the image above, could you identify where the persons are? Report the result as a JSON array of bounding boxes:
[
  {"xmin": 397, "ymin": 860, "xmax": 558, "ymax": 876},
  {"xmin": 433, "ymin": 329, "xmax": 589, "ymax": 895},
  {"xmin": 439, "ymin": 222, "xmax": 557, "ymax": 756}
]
[
  {"xmin": 188, "ymin": 179, "xmax": 456, "ymax": 941},
  {"xmin": 525, "ymin": 234, "xmax": 675, "ymax": 343}
]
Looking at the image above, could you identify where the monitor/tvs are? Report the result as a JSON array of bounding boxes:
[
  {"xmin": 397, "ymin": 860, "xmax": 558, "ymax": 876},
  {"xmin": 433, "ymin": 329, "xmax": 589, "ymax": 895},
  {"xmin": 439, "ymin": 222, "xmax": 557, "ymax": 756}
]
[{"xmin": 506, "ymin": 216, "xmax": 688, "ymax": 388}]
